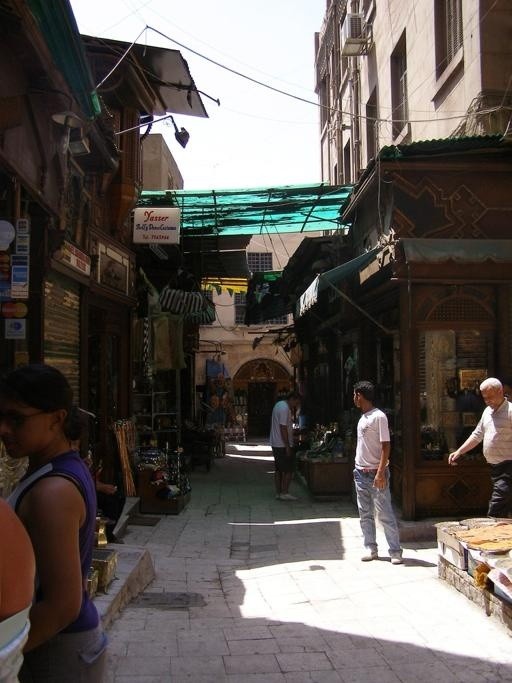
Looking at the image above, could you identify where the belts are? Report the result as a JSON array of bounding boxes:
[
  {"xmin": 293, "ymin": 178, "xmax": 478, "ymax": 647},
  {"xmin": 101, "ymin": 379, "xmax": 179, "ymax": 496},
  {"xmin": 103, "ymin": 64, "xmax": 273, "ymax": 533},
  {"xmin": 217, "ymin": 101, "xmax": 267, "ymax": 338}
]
[{"xmin": 357, "ymin": 469, "xmax": 378, "ymax": 472}]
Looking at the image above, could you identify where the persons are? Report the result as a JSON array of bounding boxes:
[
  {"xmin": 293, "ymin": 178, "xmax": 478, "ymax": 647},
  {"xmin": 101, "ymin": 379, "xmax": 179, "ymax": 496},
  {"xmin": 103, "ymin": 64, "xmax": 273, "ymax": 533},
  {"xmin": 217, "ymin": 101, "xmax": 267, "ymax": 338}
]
[
  {"xmin": 269, "ymin": 391, "xmax": 302, "ymax": 500},
  {"xmin": 0, "ymin": 364, "xmax": 108, "ymax": 683},
  {"xmin": 448, "ymin": 378, "xmax": 512, "ymax": 518},
  {"xmin": 0, "ymin": 496, "xmax": 36, "ymax": 683},
  {"xmin": 68, "ymin": 431, "xmax": 125, "ymax": 543},
  {"xmin": 352, "ymin": 380, "xmax": 403, "ymax": 563}
]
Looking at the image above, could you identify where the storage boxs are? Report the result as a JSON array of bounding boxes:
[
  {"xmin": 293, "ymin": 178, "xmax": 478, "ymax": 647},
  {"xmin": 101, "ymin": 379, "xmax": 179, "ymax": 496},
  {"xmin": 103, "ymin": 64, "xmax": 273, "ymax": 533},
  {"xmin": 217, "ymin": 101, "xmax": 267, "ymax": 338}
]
[{"xmin": 431, "ymin": 517, "xmax": 512, "ymax": 606}]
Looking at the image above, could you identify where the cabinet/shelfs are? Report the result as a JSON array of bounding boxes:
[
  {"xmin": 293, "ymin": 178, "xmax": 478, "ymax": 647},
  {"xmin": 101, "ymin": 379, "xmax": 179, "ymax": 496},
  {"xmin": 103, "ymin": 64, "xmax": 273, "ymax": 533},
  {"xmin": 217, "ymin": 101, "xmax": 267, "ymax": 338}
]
[{"xmin": 129, "ymin": 389, "xmax": 180, "ymax": 435}]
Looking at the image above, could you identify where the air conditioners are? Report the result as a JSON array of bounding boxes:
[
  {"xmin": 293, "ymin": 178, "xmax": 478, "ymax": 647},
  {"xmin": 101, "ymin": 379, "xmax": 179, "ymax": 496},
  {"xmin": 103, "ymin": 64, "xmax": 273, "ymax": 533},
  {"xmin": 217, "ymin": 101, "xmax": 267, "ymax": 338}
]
[{"xmin": 339, "ymin": 11, "xmax": 366, "ymax": 55}]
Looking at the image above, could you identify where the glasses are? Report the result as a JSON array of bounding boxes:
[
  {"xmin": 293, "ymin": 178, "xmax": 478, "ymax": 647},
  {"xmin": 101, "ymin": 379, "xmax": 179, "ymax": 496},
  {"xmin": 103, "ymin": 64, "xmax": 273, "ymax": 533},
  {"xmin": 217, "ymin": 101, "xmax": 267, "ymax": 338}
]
[{"xmin": 0, "ymin": 409, "xmax": 57, "ymax": 427}]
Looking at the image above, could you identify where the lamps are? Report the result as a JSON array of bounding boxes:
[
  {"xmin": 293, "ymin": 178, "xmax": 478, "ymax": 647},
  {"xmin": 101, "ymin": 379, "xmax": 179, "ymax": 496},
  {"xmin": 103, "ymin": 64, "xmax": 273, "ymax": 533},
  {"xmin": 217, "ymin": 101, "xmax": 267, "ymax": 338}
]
[
  {"xmin": 116, "ymin": 114, "xmax": 190, "ymax": 150},
  {"xmin": 26, "ymin": 86, "xmax": 84, "ymax": 128}
]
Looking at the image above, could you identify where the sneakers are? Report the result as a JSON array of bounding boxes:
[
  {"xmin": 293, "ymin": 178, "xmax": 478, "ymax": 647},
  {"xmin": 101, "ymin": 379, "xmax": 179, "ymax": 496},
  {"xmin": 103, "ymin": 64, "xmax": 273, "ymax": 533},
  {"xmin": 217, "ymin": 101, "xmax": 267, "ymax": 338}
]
[
  {"xmin": 360, "ymin": 549, "xmax": 378, "ymax": 560},
  {"xmin": 390, "ymin": 552, "xmax": 402, "ymax": 563},
  {"xmin": 274, "ymin": 493, "xmax": 299, "ymax": 500}
]
